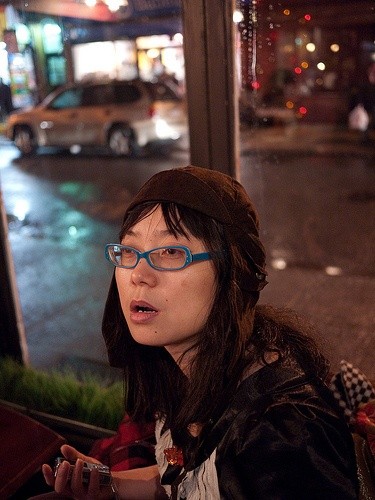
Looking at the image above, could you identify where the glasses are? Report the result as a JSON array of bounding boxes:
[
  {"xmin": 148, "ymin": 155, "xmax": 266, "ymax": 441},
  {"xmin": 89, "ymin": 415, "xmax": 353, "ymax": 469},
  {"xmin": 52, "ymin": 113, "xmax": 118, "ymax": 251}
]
[{"xmin": 104, "ymin": 243, "xmax": 215, "ymax": 271}]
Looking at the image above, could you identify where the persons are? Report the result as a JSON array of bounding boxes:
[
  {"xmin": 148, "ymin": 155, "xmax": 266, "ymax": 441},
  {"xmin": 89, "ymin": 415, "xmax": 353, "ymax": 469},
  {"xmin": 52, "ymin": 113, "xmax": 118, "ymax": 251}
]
[{"xmin": 44, "ymin": 167, "xmax": 362, "ymax": 500}]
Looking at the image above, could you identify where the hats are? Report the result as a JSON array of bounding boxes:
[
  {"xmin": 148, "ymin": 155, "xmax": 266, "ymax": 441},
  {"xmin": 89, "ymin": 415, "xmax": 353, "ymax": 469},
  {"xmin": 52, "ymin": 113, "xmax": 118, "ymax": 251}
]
[{"xmin": 122, "ymin": 165, "xmax": 261, "ymax": 229}]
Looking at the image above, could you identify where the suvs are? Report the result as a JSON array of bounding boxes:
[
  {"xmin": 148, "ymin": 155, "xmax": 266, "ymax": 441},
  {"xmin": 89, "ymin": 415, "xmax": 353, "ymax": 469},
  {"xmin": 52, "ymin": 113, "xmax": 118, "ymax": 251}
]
[{"xmin": 5, "ymin": 76, "xmax": 185, "ymax": 158}]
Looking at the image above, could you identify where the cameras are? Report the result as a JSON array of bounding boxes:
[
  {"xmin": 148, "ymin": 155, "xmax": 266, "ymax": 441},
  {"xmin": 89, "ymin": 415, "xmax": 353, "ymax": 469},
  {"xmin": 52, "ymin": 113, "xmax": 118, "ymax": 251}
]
[{"xmin": 53, "ymin": 457, "xmax": 112, "ymax": 486}]
